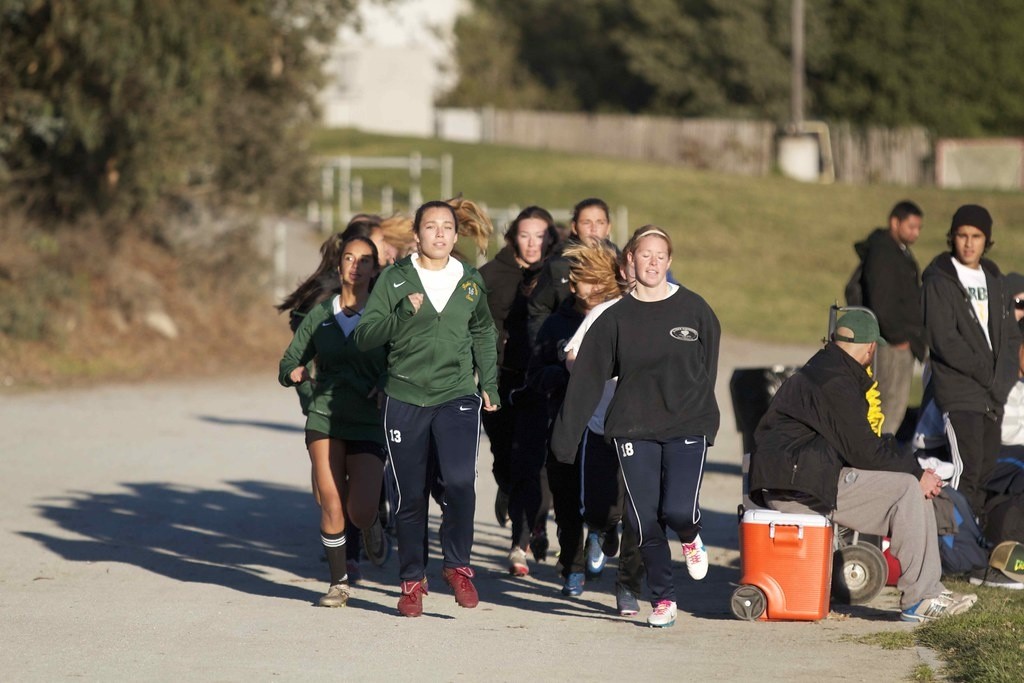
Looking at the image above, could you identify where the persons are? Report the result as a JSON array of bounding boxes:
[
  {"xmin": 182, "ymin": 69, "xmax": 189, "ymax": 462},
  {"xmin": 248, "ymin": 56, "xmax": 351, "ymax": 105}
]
[
  {"xmin": 525, "ymin": 198, "xmax": 641, "ymax": 616},
  {"xmin": 853, "ymin": 199, "xmax": 924, "ymax": 437},
  {"xmin": 920, "ymin": 205, "xmax": 1024, "ymax": 511},
  {"xmin": 278, "ymin": 236, "xmax": 393, "ymax": 608},
  {"xmin": 908, "ymin": 317, "xmax": 1024, "ymax": 589},
  {"xmin": 747, "ymin": 310, "xmax": 979, "ymax": 621},
  {"xmin": 1006, "ymin": 272, "xmax": 1024, "ymax": 382},
  {"xmin": 551, "ymin": 224, "xmax": 722, "ymax": 628},
  {"xmin": 272, "ymin": 191, "xmax": 494, "ymax": 563},
  {"xmin": 479, "ymin": 205, "xmax": 560, "ymax": 577},
  {"xmin": 354, "ymin": 200, "xmax": 502, "ymax": 618}
]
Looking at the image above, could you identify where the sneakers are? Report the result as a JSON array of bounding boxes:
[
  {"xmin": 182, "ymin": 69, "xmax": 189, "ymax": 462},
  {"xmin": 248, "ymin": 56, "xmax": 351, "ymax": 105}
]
[
  {"xmin": 398, "ymin": 575, "xmax": 429, "ymax": 618},
  {"xmin": 597, "ymin": 529, "xmax": 619, "ymax": 557},
  {"xmin": 318, "ymin": 582, "xmax": 351, "ymax": 605},
  {"xmin": 585, "ymin": 531, "xmax": 608, "ymax": 577},
  {"xmin": 554, "ymin": 561, "xmax": 566, "ymax": 583},
  {"xmin": 442, "ymin": 567, "xmax": 478, "ymax": 609},
  {"xmin": 562, "ymin": 570, "xmax": 586, "ymax": 597},
  {"xmin": 680, "ymin": 534, "xmax": 709, "ymax": 580},
  {"xmin": 616, "ymin": 588, "xmax": 640, "ymax": 617},
  {"xmin": 495, "ymin": 489, "xmax": 513, "ymax": 527},
  {"xmin": 361, "ymin": 516, "xmax": 389, "ymax": 566},
  {"xmin": 900, "ymin": 590, "xmax": 977, "ymax": 622},
  {"xmin": 530, "ymin": 534, "xmax": 549, "ymax": 562},
  {"xmin": 970, "ymin": 568, "xmax": 1024, "ymax": 590},
  {"xmin": 647, "ymin": 601, "xmax": 677, "ymax": 628},
  {"xmin": 507, "ymin": 548, "xmax": 530, "ymax": 576}
]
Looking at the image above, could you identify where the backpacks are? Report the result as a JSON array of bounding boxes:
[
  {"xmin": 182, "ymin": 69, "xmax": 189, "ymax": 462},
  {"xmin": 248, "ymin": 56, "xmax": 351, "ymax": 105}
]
[{"xmin": 931, "ymin": 484, "xmax": 992, "ymax": 578}]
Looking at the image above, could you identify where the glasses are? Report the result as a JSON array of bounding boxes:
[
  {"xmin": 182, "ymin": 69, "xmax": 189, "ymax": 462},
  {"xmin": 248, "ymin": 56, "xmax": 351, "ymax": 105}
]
[{"xmin": 1015, "ymin": 299, "xmax": 1024, "ymax": 311}]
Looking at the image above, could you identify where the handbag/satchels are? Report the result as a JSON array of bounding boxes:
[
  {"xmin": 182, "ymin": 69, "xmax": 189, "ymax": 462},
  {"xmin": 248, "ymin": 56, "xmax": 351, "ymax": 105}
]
[{"xmin": 845, "ymin": 257, "xmax": 863, "ymax": 307}]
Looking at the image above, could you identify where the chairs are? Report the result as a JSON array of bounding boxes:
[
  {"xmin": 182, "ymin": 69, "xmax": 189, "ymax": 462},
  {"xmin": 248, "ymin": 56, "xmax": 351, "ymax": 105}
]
[{"xmin": 731, "ymin": 368, "xmax": 875, "ymax": 551}]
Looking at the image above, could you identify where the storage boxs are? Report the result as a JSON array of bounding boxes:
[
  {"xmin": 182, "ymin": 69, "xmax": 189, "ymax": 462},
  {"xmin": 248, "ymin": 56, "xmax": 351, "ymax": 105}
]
[{"xmin": 730, "ymin": 504, "xmax": 834, "ymax": 621}]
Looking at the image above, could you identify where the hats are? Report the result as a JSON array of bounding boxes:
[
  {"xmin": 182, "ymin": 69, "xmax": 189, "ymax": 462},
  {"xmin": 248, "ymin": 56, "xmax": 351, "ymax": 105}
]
[
  {"xmin": 834, "ymin": 307, "xmax": 887, "ymax": 346},
  {"xmin": 1005, "ymin": 272, "xmax": 1024, "ymax": 295},
  {"xmin": 990, "ymin": 541, "xmax": 1024, "ymax": 583},
  {"xmin": 951, "ymin": 204, "xmax": 992, "ymax": 240}
]
